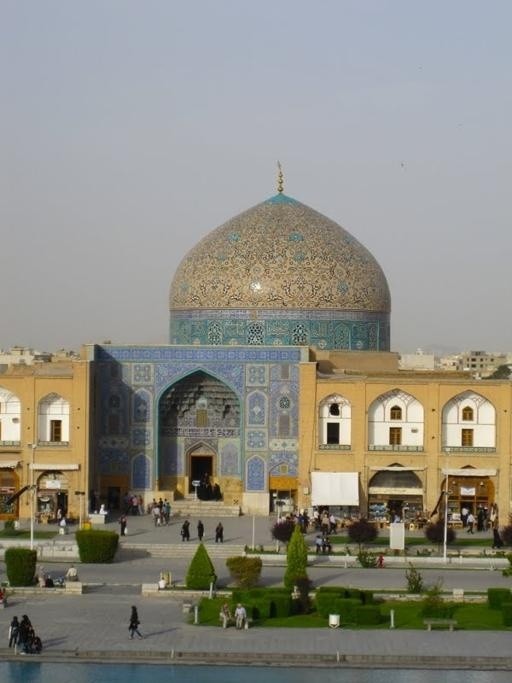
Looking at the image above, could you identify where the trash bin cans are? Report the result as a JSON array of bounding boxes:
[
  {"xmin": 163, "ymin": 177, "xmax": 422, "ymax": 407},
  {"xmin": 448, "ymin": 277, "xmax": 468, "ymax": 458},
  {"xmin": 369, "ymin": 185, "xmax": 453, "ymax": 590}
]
[{"xmin": 183, "ymin": 600, "xmax": 191, "ymax": 615}]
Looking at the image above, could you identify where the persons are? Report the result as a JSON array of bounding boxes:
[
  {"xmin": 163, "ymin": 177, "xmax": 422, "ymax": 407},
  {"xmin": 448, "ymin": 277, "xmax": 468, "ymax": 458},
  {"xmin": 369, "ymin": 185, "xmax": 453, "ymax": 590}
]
[
  {"xmin": 0, "ymin": 587, "xmax": 8, "ymax": 603},
  {"xmin": 126, "ymin": 605, "xmax": 143, "ymax": 640},
  {"xmin": 56, "ymin": 503, "xmax": 64, "ymax": 526},
  {"xmin": 275, "ymin": 506, "xmax": 337, "ymax": 554},
  {"xmin": 215, "ymin": 521, "xmax": 224, "ymax": 542},
  {"xmin": 150, "ymin": 497, "xmax": 171, "ymax": 525},
  {"xmin": 181, "ymin": 519, "xmax": 190, "ymax": 541},
  {"xmin": 197, "ymin": 520, "xmax": 204, "ymax": 540},
  {"xmin": 65, "ymin": 565, "xmax": 78, "ymax": 576},
  {"xmin": 45, "ymin": 574, "xmax": 54, "ymax": 588},
  {"xmin": 491, "ymin": 523, "xmax": 505, "ymax": 548},
  {"xmin": 197, "ymin": 471, "xmax": 222, "ymax": 501},
  {"xmin": 461, "ymin": 502, "xmax": 488, "ymax": 534},
  {"xmin": 122, "ymin": 492, "xmax": 143, "ymax": 515},
  {"xmin": 6, "ymin": 614, "xmax": 43, "ymax": 654},
  {"xmin": 233, "ymin": 602, "xmax": 247, "ymax": 630},
  {"xmin": 119, "ymin": 513, "xmax": 128, "ymax": 536},
  {"xmin": 158, "ymin": 576, "xmax": 168, "ymax": 590},
  {"xmin": 219, "ymin": 602, "xmax": 232, "ymax": 629}
]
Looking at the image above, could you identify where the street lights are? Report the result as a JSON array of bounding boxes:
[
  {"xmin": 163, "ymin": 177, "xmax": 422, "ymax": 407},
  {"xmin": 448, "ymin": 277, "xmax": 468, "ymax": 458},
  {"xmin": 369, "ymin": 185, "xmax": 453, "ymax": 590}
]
[
  {"xmin": 31, "ymin": 445, "xmax": 36, "ymax": 550},
  {"xmin": 443, "ymin": 462, "xmax": 450, "ymax": 557}
]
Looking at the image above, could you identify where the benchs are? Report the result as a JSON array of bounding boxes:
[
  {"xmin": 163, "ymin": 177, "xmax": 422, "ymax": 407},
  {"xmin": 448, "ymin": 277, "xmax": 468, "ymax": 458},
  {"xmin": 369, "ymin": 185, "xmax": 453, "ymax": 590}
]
[{"xmin": 422, "ymin": 619, "xmax": 458, "ymax": 632}]
